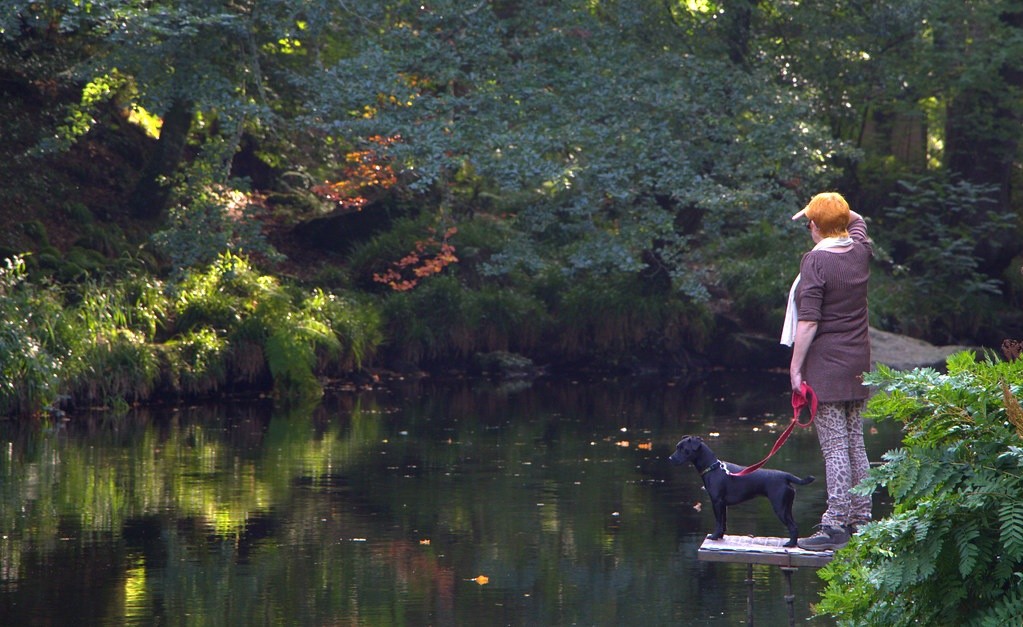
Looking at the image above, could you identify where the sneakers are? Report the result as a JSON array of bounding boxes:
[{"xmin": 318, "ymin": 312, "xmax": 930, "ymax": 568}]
[
  {"xmin": 797, "ymin": 524, "xmax": 851, "ymax": 551},
  {"xmin": 847, "ymin": 521, "xmax": 867, "ymax": 538}
]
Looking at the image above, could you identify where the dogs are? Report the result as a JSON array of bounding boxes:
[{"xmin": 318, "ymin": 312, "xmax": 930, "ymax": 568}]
[{"xmin": 668, "ymin": 434, "xmax": 816, "ymax": 547}]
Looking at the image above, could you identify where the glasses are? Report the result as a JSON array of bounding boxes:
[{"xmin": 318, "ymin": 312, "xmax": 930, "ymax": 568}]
[{"xmin": 806, "ymin": 219, "xmax": 811, "ymax": 229}]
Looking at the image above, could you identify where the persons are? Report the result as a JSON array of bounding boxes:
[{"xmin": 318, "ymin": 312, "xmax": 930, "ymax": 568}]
[{"xmin": 789, "ymin": 193, "xmax": 873, "ymax": 550}]
